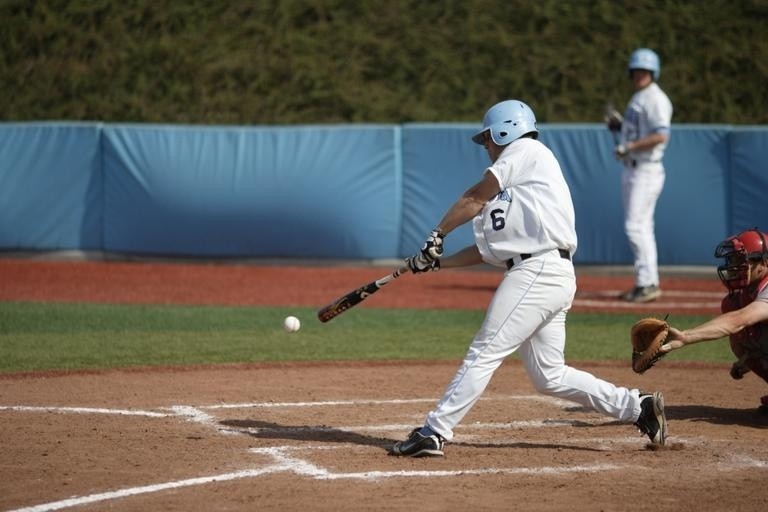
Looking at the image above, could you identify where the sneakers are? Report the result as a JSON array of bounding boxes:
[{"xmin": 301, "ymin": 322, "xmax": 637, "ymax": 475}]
[
  {"xmin": 618, "ymin": 282, "xmax": 663, "ymax": 301},
  {"xmin": 635, "ymin": 390, "xmax": 668, "ymax": 447},
  {"xmin": 389, "ymin": 424, "xmax": 448, "ymax": 459}
]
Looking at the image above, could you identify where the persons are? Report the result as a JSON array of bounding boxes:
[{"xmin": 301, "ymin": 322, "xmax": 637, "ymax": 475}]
[
  {"xmin": 605, "ymin": 49, "xmax": 673, "ymax": 303},
  {"xmin": 390, "ymin": 99, "xmax": 670, "ymax": 458},
  {"xmin": 629, "ymin": 226, "xmax": 768, "ymax": 424}
]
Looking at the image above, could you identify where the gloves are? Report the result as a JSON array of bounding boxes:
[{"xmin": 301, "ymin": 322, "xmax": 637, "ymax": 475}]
[
  {"xmin": 604, "ymin": 110, "xmax": 629, "ymax": 162},
  {"xmin": 407, "ymin": 231, "xmax": 446, "ymax": 274}
]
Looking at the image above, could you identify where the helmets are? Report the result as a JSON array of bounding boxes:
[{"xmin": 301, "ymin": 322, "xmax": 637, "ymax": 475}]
[
  {"xmin": 468, "ymin": 99, "xmax": 540, "ymax": 150},
  {"xmin": 627, "ymin": 47, "xmax": 661, "ymax": 82},
  {"xmin": 726, "ymin": 228, "xmax": 768, "ymax": 260}
]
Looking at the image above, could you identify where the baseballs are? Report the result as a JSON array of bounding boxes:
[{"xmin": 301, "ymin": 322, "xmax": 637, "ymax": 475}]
[{"xmin": 283, "ymin": 315, "xmax": 300, "ymax": 334}]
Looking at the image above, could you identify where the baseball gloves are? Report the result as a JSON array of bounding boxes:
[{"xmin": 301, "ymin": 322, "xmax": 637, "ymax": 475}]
[{"xmin": 630, "ymin": 313, "xmax": 670, "ymax": 375}]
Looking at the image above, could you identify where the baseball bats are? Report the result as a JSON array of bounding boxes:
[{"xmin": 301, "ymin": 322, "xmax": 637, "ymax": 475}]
[{"xmin": 318, "ymin": 245, "xmax": 443, "ymax": 322}]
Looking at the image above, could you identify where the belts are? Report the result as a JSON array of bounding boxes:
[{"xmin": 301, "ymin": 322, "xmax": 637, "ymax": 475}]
[{"xmin": 505, "ymin": 248, "xmax": 573, "ymax": 271}]
